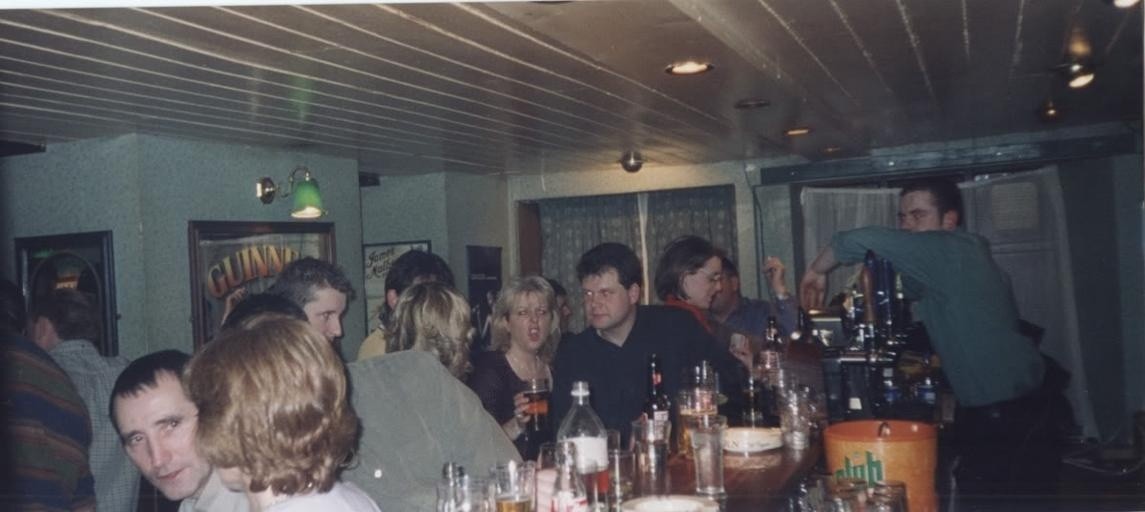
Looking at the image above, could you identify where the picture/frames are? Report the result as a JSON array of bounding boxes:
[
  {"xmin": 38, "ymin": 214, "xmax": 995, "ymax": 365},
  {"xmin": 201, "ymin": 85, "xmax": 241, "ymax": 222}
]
[
  {"xmin": 188, "ymin": 219, "xmax": 337, "ymax": 354},
  {"xmin": 16, "ymin": 229, "xmax": 119, "ymax": 359}
]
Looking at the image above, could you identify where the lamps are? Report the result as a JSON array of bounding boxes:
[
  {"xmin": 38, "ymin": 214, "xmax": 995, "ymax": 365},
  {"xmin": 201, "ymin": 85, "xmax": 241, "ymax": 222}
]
[{"xmin": 257, "ymin": 166, "xmax": 332, "ymax": 224}]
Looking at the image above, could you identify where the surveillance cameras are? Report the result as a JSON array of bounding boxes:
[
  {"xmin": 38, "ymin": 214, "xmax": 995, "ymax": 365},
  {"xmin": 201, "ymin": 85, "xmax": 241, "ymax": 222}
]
[{"xmin": 620, "ymin": 154, "xmax": 643, "ymax": 172}]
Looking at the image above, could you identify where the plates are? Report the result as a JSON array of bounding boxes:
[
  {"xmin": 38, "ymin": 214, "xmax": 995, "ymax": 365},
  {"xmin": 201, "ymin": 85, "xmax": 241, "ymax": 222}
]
[
  {"xmin": 620, "ymin": 494, "xmax": 719, "ymax": 512},
  {"xmin": 722, "ymin": 426, "xmax": 782, "ymax": 454}
]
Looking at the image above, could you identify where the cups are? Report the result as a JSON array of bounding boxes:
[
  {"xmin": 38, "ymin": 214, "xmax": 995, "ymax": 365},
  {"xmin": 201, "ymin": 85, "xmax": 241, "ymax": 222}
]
[
  {"xmin": 676, "ymin": 360, "xmax": 727, "ymax": 495},
  {"xmin": 737, "ymin": 364, "xmax": 761, "ymax": 417},
  {"xmin": 767, "ymin": 367, "xmax": 799, "ymax": 417},
  {"xmin": 777, "ymin": 387, "xmax": 813, "ymax": 451},
  {"xmin": 628, "ymin": 419, "xmax": 672, "ymax": 471},
  {"xmin": 436, "ymin": 376, "xmax": 622, "ymax": 512}
]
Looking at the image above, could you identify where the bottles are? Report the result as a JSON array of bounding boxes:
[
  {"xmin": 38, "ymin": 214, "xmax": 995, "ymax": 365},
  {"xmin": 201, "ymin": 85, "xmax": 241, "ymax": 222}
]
[
  {"xmin": 916, "ymin": 354, "xmax": 938, "ymax": 403},
  {"xmin": 757, "ymin": 314, "xmax": 784, "ymax": 367},
  {"xmin": 643, "ymin": 352, "xmax": 670, "ymax": 420},
  {"xmin": 782, "ymin": 468, "xmax": 906, "ymax": 512}
]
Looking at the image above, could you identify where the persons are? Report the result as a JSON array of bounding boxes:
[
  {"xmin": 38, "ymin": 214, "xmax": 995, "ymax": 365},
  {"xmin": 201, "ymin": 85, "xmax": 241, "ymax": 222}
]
[
  {"xmin": 25, "ymin": 283, "xmax": 144, "ymax": 512},
  {"xmin": 107, "ymin": 347, "xmax": 250, "ymax": 512},
  {"xmin": 223, "ymin": 240, "xmax": 798, "ymax": 469},
  {"xmin": 176, "ymin": 314, "xmax": 392, "ymax": 511},
  {"xmin": 798, "ymin": 175, "xmax": 1052, "ymax": 487},
  {"xmin": 0, "ymin": 275, "xmax": 98, "ymax": 512}
]
